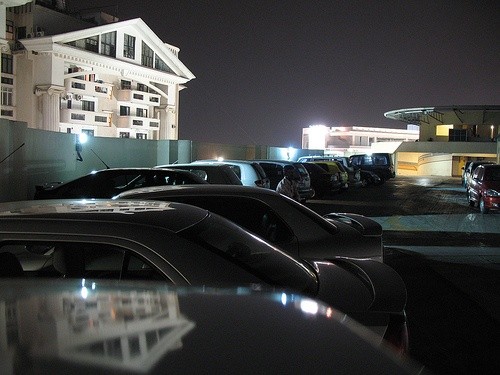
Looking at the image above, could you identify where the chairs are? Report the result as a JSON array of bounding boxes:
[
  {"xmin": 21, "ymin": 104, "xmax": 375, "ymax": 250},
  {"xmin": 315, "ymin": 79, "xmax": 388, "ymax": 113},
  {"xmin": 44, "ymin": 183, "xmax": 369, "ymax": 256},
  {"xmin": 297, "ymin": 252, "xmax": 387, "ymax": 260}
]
[
  {"xmin": 51, "ymin": 244, "xmax": 86, "ymax": 281},
  {"xmin": 142, "ymin": 174, "xmax": 166, "ymax": 187},
  {"xmin": 0, "ymin": 252, "xmax": 24, "ymax": 277}
]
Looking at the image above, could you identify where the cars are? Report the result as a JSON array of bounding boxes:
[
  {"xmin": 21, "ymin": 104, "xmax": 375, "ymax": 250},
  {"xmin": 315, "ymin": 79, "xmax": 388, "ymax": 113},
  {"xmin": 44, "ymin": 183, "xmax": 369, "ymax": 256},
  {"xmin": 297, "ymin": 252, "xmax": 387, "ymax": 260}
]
[
  {"xmin": 47, "ymin": 185, "xmax": 383, "ymax": 260},
  {"xmin": 461, "ymin": 160, "xmax": 497, "ymax": 191},
  {"xmin": 1, "ymin": 198, "xmax": 408, "ymax": 375},
  {"xmin": 39, "ymin": 153, "xmax": 398, "ymax": 212},
  {"xmin": 467, "ymin": 164, "xmax": 500, "ymax": 214}
]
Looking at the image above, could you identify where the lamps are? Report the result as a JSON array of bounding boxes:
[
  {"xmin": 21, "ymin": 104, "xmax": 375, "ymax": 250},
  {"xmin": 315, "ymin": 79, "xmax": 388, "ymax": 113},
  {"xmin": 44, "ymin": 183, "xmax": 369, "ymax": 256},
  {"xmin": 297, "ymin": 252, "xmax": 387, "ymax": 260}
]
[{"xmin": 76, "ymin": 132, "xmax": 88, "ymax": 144}]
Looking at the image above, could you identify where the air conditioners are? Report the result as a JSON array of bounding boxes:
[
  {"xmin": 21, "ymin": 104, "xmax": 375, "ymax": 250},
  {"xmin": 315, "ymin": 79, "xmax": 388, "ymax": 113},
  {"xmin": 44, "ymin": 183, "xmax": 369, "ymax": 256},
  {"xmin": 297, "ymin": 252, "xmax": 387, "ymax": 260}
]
[
  {"xmin": 36, "ymin": 32, "xmax": 45, "ymax": 37},
  {"xmin": 26, "ymin": 33, "xmax": 34, "ymax": 38}
]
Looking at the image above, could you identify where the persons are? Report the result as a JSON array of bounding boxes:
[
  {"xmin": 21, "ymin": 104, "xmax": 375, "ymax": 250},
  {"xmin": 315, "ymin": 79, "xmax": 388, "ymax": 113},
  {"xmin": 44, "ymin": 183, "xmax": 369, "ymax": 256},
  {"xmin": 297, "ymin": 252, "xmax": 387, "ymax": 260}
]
[{"xmin": 275, "ymin": 164, "xmax": 301, "ymax": 203}]
